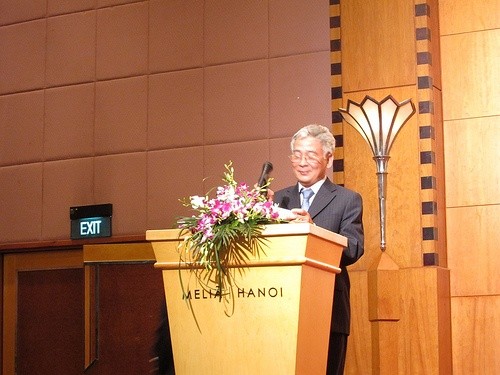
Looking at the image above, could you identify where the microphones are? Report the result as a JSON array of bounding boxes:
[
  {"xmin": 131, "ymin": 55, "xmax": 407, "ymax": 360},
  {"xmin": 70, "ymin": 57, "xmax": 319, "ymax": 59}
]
[
  {"xmin": 257, "ymin": 160, "xmax": 273, "ymax": 186},
  {"xmin": 281, "ymin": 196, "xmax": 289, "ymax": 209}
]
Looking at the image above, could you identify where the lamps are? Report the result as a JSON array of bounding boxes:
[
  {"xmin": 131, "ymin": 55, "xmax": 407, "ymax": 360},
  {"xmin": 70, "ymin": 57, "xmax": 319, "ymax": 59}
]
[{"xmin": 337, "ymin": 97, "xmax": 416, "ymax": 250}]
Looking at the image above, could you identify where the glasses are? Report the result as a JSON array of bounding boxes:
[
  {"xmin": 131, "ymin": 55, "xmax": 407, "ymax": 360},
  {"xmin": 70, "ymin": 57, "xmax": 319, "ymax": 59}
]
[{"xmin": 288, "ymin": 154, "xmax": 323, "ymax": 162}]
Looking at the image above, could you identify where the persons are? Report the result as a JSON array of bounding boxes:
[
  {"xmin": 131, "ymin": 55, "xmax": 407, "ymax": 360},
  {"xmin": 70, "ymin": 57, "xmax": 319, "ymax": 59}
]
[{"xmin": 267, "ymin": 125, "xmax": 364, "ymax": 375}]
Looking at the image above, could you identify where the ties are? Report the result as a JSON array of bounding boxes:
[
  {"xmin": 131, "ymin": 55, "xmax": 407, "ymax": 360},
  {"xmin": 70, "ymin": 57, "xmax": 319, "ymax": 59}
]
[{"xmin": 301, "ymin": 189, "xmax": 314, "ymax": 211}]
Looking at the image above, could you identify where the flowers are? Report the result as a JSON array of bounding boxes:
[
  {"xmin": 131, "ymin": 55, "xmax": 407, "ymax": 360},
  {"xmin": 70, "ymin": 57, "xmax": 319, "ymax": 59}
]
[{"xmin": 172, "ymin": 160, "xmax": 305, "ymax": 334}]
